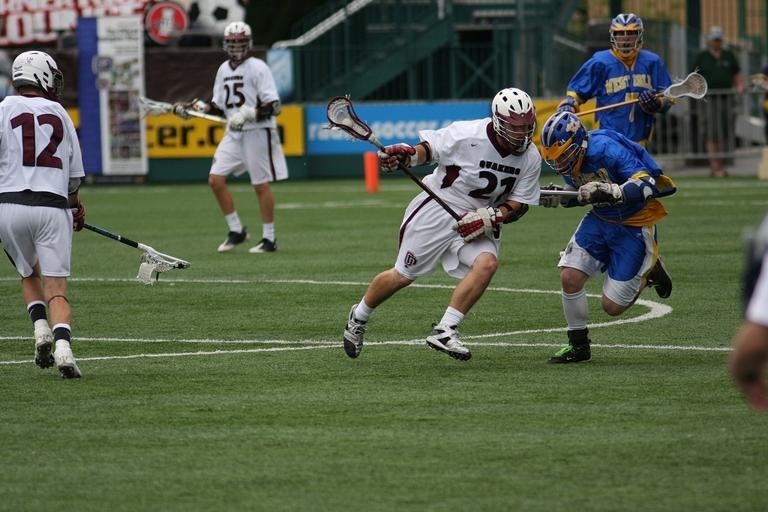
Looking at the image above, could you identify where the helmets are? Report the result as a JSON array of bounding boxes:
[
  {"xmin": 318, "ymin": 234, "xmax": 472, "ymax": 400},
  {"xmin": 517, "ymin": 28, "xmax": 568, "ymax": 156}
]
[
  {"xmin": 608, "ymin": 12, "xmax": 644, "ymax": 62},
  {"xmin": 490, "ymin": 87, "xmax": 538, "ymax": 156},
  {"xmin": 221, "ymin": 20, "xmax": 255, "ymax": 60},
  {"xmin": 10, "ymin": 48, "xmax": 65, "ymax": 99},
  {"xmin": 539, "ymin": 109, "xmax": 588, "ymax": 178}
]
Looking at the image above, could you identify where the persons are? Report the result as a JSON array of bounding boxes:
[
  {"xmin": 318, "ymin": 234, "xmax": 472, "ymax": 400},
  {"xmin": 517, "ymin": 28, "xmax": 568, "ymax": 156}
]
[
  {"xmin": 173, "ymin": 20, "xmax": 289, "ymax": 254},
  {"xmin": 0, "ymin": 51, "xmax": 85, "ymax": 377},
  {"xmin": 727, "ymin": 249, "xmax": 768, "ymax": 408},
  {"xmin": 0, "ymin": 49, "xmax": 14, "ymax": 100},
  {"xmin": 539, "ymin": 110, "xmax": 672, "ymax": 363},
  {"xmin": 557, "ymin": 13, "xmax": 674, "ymax": 147},
  {"xmin": 688, "ymin": 26, "xmax": 746, "ymax": 176},
  {"xmin": 343, "ymin": 86, "xmax": 542, "ymax": 357},
  {"xmin": 758, "ymin": 62, "xmax": 768, "ymax": 179}
]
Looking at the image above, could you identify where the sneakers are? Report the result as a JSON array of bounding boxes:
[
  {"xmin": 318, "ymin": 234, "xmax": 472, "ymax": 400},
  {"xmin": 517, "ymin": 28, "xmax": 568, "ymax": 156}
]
[
  {"xmin": 646, "ymin": 257, "xmax": 674, "ymax": 300},
  {"xmin": 342, "ymin": 303, "xmax": 366, "ymax": 361},
  {"xmin": 52, "ymin": 345, "xmax": 84, "ymax": 380},
  {"xmin": 216, "ymin": 227, "xmax": 249, "ymax": 253},
  {"xmin": 247, "ymin": 236, "xmax": 281, "ymax": 254},
  {"xmin": 544, "ymin": 343, "xmax": 594, "ymax": 365},
  {"xmin": 424, "ymin": 323, "xmax": 475, "ymax": 363},
  {"xmin": 31, "ymin": 326, "xmax": 56, "ymax": 373}
]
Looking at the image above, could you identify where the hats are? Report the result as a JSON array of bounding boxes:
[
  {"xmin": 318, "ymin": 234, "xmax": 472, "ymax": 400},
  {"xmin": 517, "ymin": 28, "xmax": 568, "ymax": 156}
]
[{"xmin": 708, "ymin": 26, "xmax": 723, "ymax": 41}]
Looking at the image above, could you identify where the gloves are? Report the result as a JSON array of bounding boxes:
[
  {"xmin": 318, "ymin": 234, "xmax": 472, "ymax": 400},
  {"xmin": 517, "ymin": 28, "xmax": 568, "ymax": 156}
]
[
  {"xmin": 556, "ymin": 102, "xmax": 579, "ymax": 117},
  {"xmin": 635, "ymin": 87, "xmax": 668, "ymax": 115},
  {"xmin": 170, "ymin": 97, "xmax": 208, "ymax": 119},
  {"xmin": 377, "ymin": 141, "xmax": 419, "ymax": 174},
  {"xmin": 72, "ymin": 201, "xmax": 86, "ymax": 232},
  {"xmin": 452, "ymin": 205, "xmax": 502, "ymax": 243},
  {"xmin": 576, "ymin": 179, "xmax": 625, "ymax": 209},
  {"xmin": 227, "ymin": 104, "xmax": 255, "ymax": 132}
]
